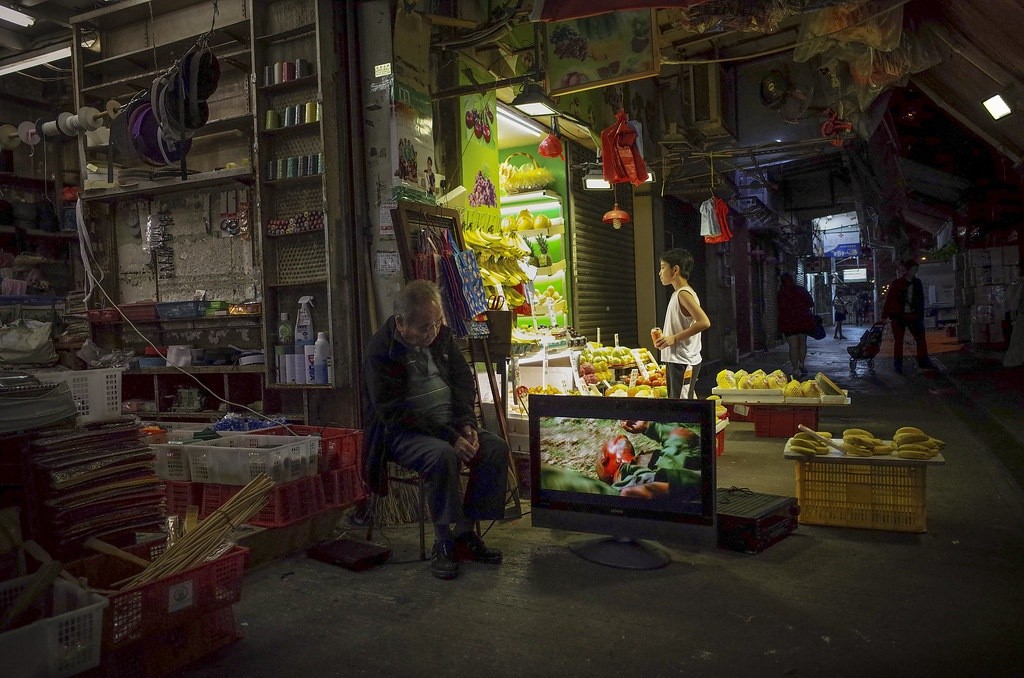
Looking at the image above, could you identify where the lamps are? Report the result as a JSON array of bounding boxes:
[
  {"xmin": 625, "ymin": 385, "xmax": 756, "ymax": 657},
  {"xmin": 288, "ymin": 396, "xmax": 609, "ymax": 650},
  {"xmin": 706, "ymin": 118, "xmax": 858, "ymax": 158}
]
[{"xmin": 980, "ymin": 81, "xmax": 1024, "ymax": 125}]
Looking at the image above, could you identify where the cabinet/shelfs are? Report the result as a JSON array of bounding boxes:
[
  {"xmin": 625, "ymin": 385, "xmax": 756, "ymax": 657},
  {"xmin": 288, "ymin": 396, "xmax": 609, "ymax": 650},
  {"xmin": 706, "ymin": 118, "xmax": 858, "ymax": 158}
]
[
  {"xmin": 500, "ymin": 190, "xmax": 573, "ymax": 351},
  {"xmin": 69, "ymin": 0, "xmax": 363, "ymax": 435},
  {"xmin": 0, "ymin": 172, "xmax": 120, "ymax": 363}
]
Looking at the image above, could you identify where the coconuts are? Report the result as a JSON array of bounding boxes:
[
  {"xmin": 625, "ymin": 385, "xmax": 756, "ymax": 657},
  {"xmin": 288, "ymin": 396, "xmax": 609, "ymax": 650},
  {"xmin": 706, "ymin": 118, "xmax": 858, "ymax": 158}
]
[{"xmin": 500, "ymin": 209, "xmax": 551, "ymax": 230}]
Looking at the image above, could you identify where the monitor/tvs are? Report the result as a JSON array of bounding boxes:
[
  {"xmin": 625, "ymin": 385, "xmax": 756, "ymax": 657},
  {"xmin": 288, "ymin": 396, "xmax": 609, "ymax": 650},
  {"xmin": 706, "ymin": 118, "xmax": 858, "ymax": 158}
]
[{"xmin": 526, "ymin": 394, "xmax": 717, "ymax": 568}]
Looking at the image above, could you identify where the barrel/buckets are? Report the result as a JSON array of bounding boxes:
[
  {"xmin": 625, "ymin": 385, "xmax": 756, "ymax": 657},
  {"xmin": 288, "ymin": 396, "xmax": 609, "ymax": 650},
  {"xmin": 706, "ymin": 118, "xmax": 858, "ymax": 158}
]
[{"xmin": 13, "ymin": 201, "xmax": 77, "ymax": 232}]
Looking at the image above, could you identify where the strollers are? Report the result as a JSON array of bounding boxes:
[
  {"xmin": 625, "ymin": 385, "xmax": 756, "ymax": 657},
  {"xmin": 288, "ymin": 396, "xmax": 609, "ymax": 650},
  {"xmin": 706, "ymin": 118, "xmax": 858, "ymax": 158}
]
[{"xmin": 848, "ymin": 322, "xmax": 887, "ymax": 371}]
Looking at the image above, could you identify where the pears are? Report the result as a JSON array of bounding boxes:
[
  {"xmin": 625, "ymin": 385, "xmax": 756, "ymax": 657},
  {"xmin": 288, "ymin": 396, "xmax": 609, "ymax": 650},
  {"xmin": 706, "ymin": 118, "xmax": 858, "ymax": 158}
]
[
  {"xmin": 580, "ymin": 341, "xmax": 649, "ymax": 366},
  {"xmin": 543, "ymin": 286, "xmax": 560, "ymax": 300}
]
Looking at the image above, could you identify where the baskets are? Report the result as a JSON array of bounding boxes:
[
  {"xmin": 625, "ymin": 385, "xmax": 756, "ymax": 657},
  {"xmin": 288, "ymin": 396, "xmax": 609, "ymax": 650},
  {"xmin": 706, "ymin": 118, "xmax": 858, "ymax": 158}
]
[
  {"xmin": 0, "ymin": 538, "xmax": 248, "ymax": 678},
  {"xmin": 119, "ymin": 304, "xmax": 158, "ymax": 320},
  {"xmin": 86, "ymin": 307, "xmax": 119, "ymax": 323},
  {"xmin": 158, "ymin": 301, "xmax": 208, "ymax": 319},
  {"xmin": 142, "ymin": 422, "xmax": 372, "ymax": 527}
]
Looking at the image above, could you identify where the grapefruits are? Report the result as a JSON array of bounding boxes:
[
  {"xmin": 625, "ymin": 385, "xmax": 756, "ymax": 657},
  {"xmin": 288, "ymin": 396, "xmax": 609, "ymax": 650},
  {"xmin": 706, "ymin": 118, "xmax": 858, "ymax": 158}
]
[{"xmin": 716, "ymin": 369, "xmax": 821, "ymax": 398}]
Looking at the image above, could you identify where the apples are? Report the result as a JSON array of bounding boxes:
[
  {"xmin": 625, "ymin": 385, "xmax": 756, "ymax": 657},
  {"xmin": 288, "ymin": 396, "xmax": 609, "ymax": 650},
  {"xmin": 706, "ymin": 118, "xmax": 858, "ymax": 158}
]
[{"xmin": 625, "ymin": 362, "xmax": 668, "ymax": 387}]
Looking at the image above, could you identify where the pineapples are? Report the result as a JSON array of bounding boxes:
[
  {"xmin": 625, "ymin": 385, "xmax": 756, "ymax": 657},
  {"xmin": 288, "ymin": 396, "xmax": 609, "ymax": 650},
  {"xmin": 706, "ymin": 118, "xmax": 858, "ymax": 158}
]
[{"xmin": 523, "ymin": 234, "xmax": 552, "ymax": 267}]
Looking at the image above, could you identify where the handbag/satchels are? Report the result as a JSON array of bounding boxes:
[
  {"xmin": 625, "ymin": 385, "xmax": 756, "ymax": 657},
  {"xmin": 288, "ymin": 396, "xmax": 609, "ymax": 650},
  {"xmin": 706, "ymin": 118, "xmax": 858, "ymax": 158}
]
[
  {"xmin": 806, "ymin": 303, "xmax": 825, "ymax": 340},
  {"xmin": 413, "ymin": 227, "xmax": 490, "ymax": 339}
]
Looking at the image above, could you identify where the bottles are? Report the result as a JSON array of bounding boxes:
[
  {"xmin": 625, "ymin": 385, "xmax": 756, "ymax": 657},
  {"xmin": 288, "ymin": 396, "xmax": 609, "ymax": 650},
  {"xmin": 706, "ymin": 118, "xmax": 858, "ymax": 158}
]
[
  {"xmin": 277, "ymin": 313, "xmax": 294, "ymax": 344},
  {"xmin": 314, "ymin": 331, "xmax": 328, "ymax": 384}
]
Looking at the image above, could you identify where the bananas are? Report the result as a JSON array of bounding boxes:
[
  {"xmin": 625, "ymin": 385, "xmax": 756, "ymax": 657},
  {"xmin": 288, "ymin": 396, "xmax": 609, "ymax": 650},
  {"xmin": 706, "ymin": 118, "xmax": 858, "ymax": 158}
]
[
  {"xmin": 511, "ymin": 326, "xmax": 541, "ymax": 345},
  {"xmin": 460, "ymin": 222, "xmax": 532, "ymax": 306},
  {"xmin": 787, "ymin": 425, "xmax": 946, "ymax": 460}
]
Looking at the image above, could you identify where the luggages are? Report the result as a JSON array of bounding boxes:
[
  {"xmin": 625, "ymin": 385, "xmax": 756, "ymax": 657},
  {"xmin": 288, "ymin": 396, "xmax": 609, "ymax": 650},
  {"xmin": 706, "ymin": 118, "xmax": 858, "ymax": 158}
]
[{"xmin": 846, "ymin": 321, "xmax": 887, "ymax": 373}]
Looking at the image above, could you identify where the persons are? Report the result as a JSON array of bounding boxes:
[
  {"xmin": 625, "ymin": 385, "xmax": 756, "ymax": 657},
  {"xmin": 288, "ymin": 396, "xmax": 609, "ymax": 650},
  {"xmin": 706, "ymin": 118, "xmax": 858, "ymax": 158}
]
[
  {"xmin": 778, "ymin": 272, "xmax": 814, "ymax": 378},
  {"xmin": 832, "ymin": 289, "xmax": 848, "ymax": 341},
  {"xmin": 654, "ymin": 249, "xmax": 712, "ymax": 401},
  {"xmin": 851, "ymin": 297, "xmax": 868, "ymax": 327},
  {"xmin": 359, "ymin": 276, "xmax": 508, "ymax": 580},
  {"xmin": 880, "ymin": 260, "xmax": 940, "ymax": 373}
]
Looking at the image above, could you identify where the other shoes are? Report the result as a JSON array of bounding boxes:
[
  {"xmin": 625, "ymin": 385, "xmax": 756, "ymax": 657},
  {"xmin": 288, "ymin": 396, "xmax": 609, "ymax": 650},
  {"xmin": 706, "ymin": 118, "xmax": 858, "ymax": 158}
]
[
  {"xmin": 791, "ymin": 368, "xmax": 801, "ymax": 378},
  {"xmin": 834, "ymin": 336, "xmax": 838, "ymax": 338},
  {"xmin": 895, "ymin": 366, "xmax": 903, "ymax": 373},
  {"xmin": 840, "ymin": 335, "xmax": 847, "ymax": 339},
  {"xmin": 798, "ymin": 364, "xmax": 808, "ymax": 373},
  {"xmin": 919, "ymin": 363, "xmax": 937, "ymax": 369}
]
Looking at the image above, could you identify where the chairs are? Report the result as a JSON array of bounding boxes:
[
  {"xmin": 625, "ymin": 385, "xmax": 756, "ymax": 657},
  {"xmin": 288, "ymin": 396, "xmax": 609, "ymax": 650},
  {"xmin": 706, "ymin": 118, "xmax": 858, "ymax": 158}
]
[{"xmin": 367, "ymin": 455, "xmax": 481, "ymax": 559}]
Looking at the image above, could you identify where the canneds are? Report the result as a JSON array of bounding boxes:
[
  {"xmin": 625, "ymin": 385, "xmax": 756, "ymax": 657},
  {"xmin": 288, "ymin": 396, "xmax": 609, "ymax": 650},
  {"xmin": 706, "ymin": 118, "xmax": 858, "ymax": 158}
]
[{"xmin": 650, "ymin": 327, "xmax": 663, "ymax": 348}]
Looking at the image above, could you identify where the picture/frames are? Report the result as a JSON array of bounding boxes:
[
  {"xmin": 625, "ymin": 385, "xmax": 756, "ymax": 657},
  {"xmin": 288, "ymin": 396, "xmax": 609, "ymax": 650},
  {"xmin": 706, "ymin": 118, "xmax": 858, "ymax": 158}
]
[{"xmin": 542, "ymin": 7, "xmax": 662, "ymax": 98}]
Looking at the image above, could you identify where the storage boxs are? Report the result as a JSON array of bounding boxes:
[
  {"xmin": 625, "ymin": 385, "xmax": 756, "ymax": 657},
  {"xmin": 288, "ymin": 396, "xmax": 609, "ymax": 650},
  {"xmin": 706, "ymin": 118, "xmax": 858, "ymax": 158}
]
[
  {"xmin": 477, "ymin": 350, "xmax": 583, "ymax": 456},
  {"xmin": 795, "ymin": 458, "xmax": 928, "ymax": 532},
  {"xmin": 716, "ymin": 404, "xmax": 821, "ymax": 459},
  {"xmin": 0, "ymin": 530, "xmax": 250, "ymax": 678},
  {"xmin": 140, "ymin": 419, "xmax": 375, "ymax": 527},
  {"xmin": 30, "ymin": 367, "xmax": 126, "ymax": 424}
]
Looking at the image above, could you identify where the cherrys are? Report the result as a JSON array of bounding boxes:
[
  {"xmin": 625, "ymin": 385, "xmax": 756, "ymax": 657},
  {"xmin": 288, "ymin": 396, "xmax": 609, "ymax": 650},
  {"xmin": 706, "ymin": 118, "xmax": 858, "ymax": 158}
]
[{"xmin": 466, "ymin": 106, "xmax": 494, "ymax": 143}]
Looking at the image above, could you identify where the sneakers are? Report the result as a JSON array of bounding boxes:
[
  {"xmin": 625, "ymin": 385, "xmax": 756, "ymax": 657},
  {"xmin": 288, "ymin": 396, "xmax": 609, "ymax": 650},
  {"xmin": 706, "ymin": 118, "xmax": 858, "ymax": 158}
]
[
  {"xmin": 432, "ymin": 540, "xmax": 459, "ymax": 579},
  {"xmin": 449, "ymin": 531, "xmax": 504, "ymax": 564}
]
